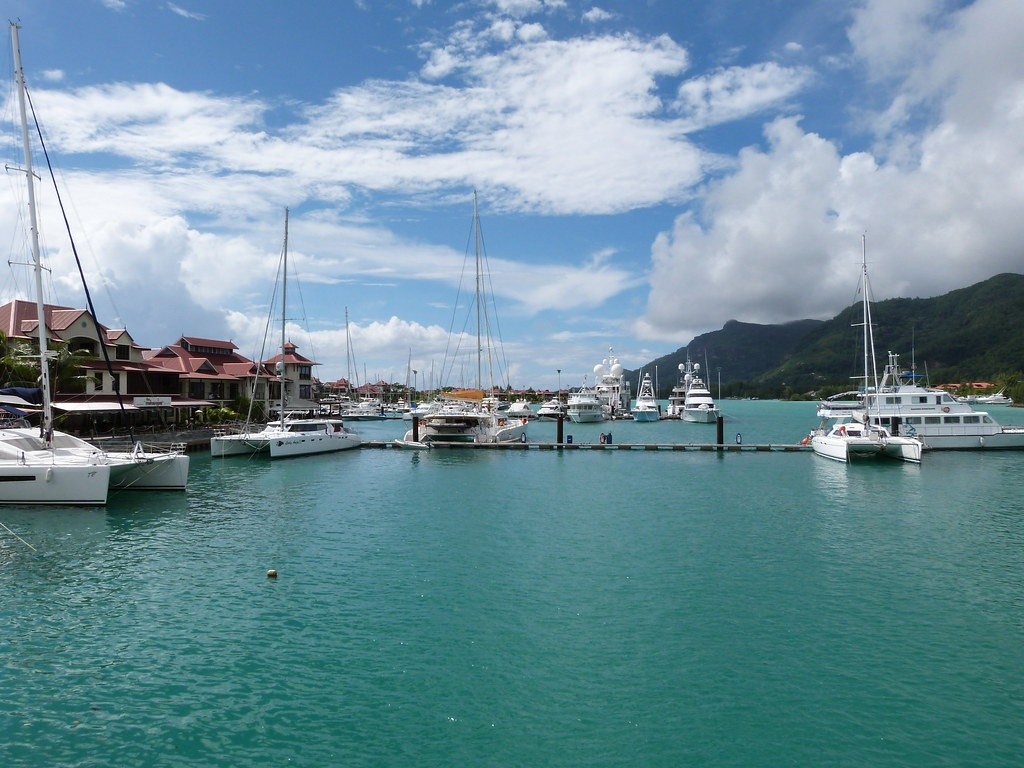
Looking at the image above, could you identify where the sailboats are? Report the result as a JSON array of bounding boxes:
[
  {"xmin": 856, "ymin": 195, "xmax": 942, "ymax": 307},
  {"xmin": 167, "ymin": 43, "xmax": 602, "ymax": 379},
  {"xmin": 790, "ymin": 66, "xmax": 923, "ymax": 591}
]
[
  {"xmin": 321, "ymin": 306, "xmax": 429, "ymax": 420},
  {"xmin": 0, "ymin": 15, "xmax": 191, "ymax": 505},
  {"xmin": 811, "ymin": 234, "xmax": 925, "ymax": 469},
  {"xmin": 405, "ymin": 189, "xmax": 528, "ymax": 443},
  {"xmin": 210, "ymin": 208, "xmax": 363, "ymax": 459}
]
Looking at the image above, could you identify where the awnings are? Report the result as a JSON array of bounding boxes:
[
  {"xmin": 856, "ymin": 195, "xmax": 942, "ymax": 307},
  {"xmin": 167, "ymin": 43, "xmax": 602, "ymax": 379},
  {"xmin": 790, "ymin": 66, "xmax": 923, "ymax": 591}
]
[{"xmin": 50, "ymin": 402, "xmax": 139, "ymax": 420}]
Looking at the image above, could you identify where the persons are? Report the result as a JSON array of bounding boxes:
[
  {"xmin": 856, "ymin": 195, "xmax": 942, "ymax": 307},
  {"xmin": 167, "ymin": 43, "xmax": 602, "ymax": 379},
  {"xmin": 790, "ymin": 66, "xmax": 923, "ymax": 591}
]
[{"xmin": 840, "ymin": 426, "xmax": 848, "ymax": 436}]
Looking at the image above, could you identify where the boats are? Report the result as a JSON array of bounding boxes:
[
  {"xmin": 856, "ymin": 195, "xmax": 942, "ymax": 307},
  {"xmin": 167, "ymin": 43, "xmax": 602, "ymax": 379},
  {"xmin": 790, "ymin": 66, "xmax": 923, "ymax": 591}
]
[
  {"xmin": 664, "ymin": 347, "xmax": 720, "ymax": 423},
  {"xmin": 865, "ymin": 326, "xmax": 1024, "ymax": 453},
  {"xmin": 536, "ymin": 357, "xmax": 633, "ymax": 424},
  {"xmin": 506, "ymin": 400, "xmax": 534, "ymax": 416},
  {"xmin": 820, "ymin": 407, "xmax": 862, "ymax": 418},
  {"xmin": 815, "ymin": 387, "xmax": 868, "ymax": 410},
  {"xmin": 631, "ymin": 365, "xmax": 660, "ymax": 421}
]
